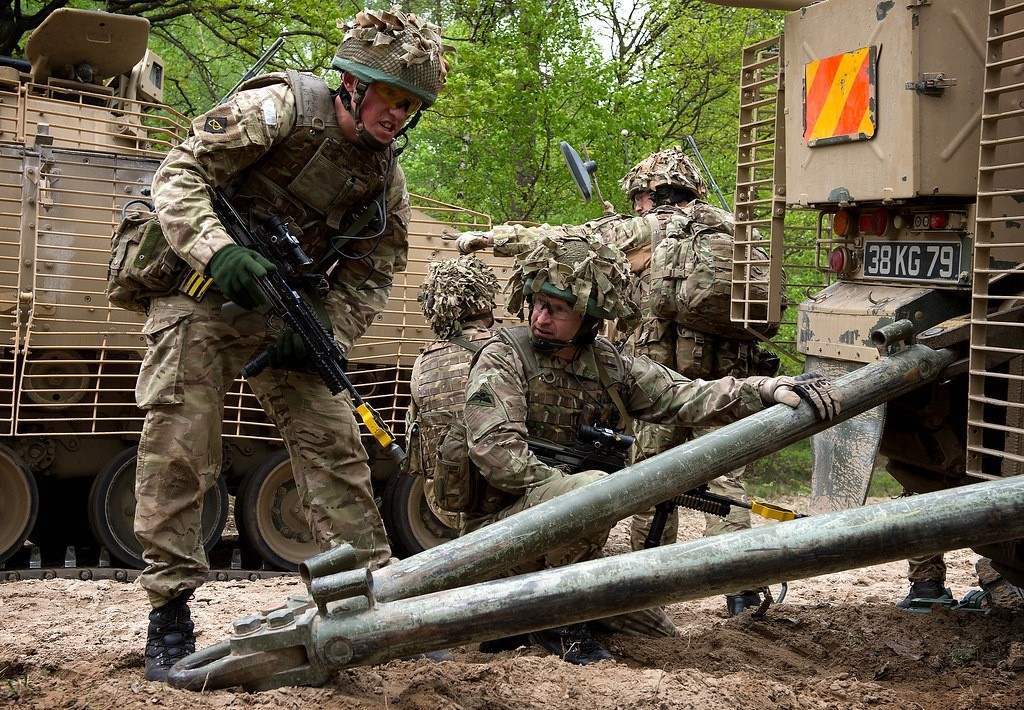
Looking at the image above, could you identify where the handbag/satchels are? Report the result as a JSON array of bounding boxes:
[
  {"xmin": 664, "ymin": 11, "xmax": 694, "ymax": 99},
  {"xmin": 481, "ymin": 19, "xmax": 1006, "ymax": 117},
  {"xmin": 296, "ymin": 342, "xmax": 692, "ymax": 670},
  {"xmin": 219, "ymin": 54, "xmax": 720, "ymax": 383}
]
[
  {"xmin": 431, "ymin": 426, "xmax": 492, "ymax": 514},
  {"xmin": 105, "ymin": 215, "xmax": 188, "ymax": 312}
]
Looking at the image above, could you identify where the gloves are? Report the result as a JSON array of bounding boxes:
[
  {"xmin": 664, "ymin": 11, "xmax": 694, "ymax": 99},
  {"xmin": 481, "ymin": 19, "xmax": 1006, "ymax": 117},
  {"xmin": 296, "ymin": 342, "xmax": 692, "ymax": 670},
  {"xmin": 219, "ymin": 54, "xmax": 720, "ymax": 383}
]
[
  {"xmin": 267, "ymin": 312, "xmax": 334, "ymax": 374},
  {"xmin": 761, "ymin": 369, "xmax": 843, "ymax": 422},
  {"xmin": 455, "ymin": 230, "xmax": 485, "ymax": 255},
  {"xmin": 209, "ymin": 244, "xmax": 277, "ymax": 311}
]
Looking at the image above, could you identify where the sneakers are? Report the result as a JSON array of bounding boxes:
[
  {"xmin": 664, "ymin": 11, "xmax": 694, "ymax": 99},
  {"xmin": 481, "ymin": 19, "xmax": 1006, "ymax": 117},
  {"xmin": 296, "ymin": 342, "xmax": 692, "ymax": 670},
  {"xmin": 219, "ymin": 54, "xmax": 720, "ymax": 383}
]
[{"xmin": 530, "ymin": 624, "xmax": 615, "ymax": 667}]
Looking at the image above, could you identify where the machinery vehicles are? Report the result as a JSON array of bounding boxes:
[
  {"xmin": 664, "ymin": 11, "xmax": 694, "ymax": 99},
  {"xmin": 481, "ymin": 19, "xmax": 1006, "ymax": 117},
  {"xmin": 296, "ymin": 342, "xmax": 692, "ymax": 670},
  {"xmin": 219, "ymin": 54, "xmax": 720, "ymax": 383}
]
[
  {"xmin": 704, "ymin": 0, "xmax": 1023, "ymax": 581},
  {"xmin": 0, "ymin": 9, "xmax": 619, "ymax": 578}
]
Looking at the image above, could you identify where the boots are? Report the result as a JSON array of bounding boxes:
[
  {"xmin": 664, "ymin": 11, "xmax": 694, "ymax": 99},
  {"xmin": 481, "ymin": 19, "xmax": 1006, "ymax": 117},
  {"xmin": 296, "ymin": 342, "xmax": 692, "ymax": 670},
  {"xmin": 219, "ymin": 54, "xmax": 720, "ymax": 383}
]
[
  {"xmin": 406, "ymin": 649, "xmax": 454, "ymax": 662},
  {"xmin": 144, "ymin": 602, "xmax": 196, "ymax": 682}
]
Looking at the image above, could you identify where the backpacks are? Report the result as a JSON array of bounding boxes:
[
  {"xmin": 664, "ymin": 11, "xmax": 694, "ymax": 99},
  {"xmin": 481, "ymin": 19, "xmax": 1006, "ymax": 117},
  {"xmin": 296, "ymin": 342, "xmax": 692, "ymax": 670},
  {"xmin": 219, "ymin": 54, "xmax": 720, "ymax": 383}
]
[{"xmin": 650, "ymin": 203, "xmax": 790, "ymax": 346}]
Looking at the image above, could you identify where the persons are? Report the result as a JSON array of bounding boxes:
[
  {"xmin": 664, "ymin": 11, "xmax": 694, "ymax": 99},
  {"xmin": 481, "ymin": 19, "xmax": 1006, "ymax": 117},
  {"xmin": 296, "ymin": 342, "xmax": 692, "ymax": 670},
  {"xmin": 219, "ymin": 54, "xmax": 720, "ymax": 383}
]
[
  {"xmin": 401, "ymin": 148, "xmax": 842, "ymax": 664},
  {"xmin": 897, "ymin": 550, "xmax": 1024, "ymax": 620},
  {"xmin": 107, "ymin": 9, "xmax": 444, "ymax": 683}
]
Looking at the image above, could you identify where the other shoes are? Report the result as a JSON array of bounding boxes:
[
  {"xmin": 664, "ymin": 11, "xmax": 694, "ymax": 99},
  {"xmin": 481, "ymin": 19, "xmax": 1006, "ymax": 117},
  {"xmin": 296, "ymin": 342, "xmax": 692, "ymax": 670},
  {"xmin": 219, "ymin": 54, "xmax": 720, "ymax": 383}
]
[
  {"xmin": 895, "ymin": 580, "xmax": 949, "ymax": 610},
  {"xmin": 727, "ymin": 591, "xmax": 762, "ymax": 618}
]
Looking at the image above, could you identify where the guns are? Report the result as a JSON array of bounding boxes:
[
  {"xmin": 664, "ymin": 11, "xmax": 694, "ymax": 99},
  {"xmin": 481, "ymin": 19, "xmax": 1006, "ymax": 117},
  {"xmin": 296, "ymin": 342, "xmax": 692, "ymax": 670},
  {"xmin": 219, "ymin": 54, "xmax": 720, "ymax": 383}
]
[
  {"xmin": 527, "ymin": 425, "xmax": 811, "ymax": 521},
  {"xmin": 176, "ymin": 187, "xmax": 408, "ymax": 466}
]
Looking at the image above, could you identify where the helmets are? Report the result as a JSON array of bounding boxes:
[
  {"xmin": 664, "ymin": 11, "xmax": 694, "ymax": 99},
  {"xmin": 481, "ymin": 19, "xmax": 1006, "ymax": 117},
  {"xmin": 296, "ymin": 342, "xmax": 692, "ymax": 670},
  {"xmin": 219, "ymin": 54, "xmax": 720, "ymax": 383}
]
[
  {"xmin": 626, "ymin": 147, "xmax": 707, "ymax": 200},
  {"xmin": 523, "ymin": 238, "xmax": 627, "ymax": 319},
  {"xmin": 330, "ymin": 8, "xmax": 449, "ymax": 110},
  {"xmin": 417, "ymin": 257, "xmax": 499, "ymax": 322}
]
